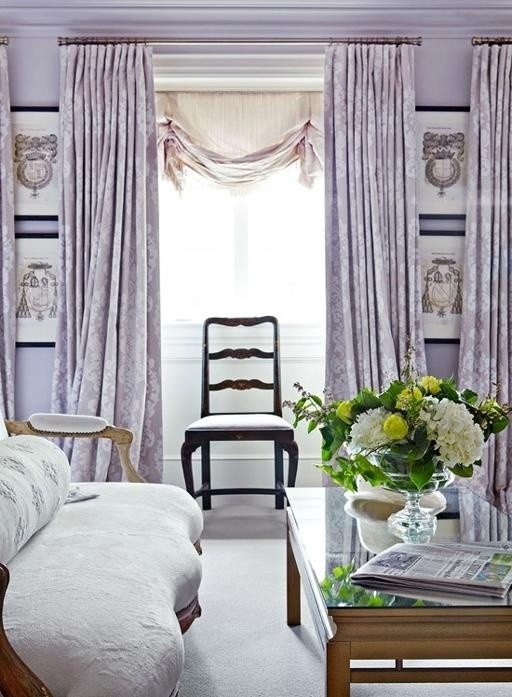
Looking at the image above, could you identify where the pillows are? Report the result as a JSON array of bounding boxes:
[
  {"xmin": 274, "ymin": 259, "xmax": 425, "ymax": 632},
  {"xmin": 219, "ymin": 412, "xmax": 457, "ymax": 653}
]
[{"xmin": 0, "ymin": 435, "xmax": 70, "ymax": 565}]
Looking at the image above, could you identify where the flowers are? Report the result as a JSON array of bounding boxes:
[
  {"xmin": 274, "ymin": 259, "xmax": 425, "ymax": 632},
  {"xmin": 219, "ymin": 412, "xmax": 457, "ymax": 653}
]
[{"xmin": 283, "ymin": 334, "xmax": 512, "ymax": 528}]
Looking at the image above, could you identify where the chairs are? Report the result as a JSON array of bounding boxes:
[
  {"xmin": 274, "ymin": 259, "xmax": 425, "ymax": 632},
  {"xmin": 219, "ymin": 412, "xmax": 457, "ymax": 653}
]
[{"xmin": 181, "ymin": 316, "xmax": 299, "ymax": 510}]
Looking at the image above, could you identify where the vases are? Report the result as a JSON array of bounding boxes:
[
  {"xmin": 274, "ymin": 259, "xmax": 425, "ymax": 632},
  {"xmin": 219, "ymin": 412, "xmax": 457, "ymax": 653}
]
[{"xmin": 368, "ymin": 453, "xmax": 451, "ymax": 544}]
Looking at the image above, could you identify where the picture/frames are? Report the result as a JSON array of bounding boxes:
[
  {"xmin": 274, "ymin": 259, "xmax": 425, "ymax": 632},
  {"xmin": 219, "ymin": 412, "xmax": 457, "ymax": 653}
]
[
  {"xmin": 10, "ymin": 106, "xmax": 60, "ymax": 221},
  {"xmin": 15, "ymin": 233, "xmax": 59, "ymax": 347},
  {"xmin": 415, "ymin": 106, "xmax": 470, "ymax": 219},
  {"xmin": 420, "ymin": 230, "xmax": 466, "ymax": 344}
]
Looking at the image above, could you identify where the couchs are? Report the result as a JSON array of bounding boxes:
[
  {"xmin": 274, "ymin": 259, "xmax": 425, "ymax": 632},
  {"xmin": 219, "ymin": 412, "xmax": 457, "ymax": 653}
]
[{"xmin": 0, "ymin": 409, "xmax": 204, "ymax": 697}]
